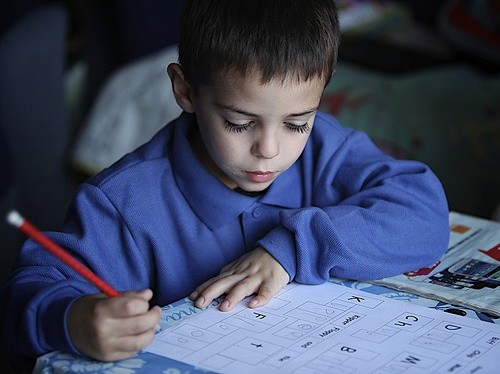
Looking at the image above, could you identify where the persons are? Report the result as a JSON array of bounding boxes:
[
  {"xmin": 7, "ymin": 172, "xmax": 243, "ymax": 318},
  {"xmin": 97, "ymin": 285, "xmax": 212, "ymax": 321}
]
[{"xmin": 0, "ymin": 0, "xmax": 453, "ymax": 366}]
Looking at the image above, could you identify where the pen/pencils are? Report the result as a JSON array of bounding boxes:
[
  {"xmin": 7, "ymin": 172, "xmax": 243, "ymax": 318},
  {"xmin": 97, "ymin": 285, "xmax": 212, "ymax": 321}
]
[{"xmin": 7, "ymin": 211, "xmax": 121, "ymax": 299}]
